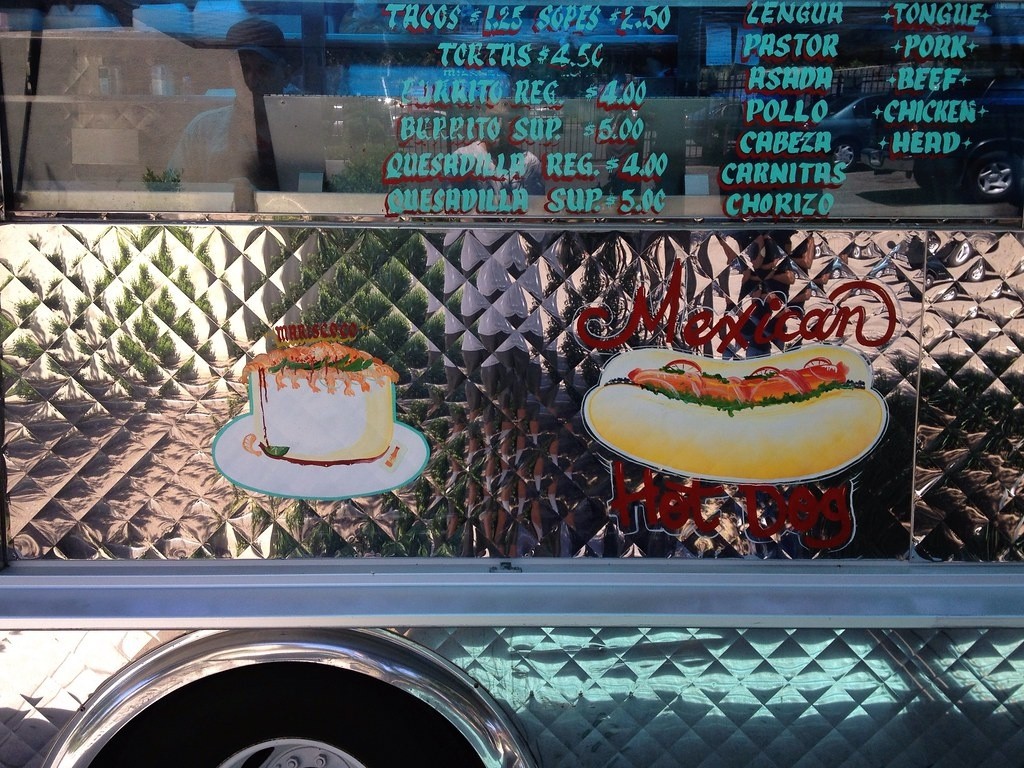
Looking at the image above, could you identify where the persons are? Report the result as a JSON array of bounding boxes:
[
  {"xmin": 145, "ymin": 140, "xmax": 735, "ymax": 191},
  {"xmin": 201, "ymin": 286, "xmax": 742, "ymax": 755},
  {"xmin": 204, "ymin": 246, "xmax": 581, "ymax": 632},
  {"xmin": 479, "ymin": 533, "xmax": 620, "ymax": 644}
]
[
  {"xmin": 448, "ymin": 97, "xmax": 546, "ymax": 196},
  {"xmin": 166, "ymin": 18, "xmax": 308, "ymax": 191}
]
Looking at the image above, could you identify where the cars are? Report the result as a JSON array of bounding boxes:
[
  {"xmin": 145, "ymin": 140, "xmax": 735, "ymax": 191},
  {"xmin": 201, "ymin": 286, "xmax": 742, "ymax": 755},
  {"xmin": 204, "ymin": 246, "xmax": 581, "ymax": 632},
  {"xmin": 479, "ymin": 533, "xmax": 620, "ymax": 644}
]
[
  {"xmin": 331, "ymin": 97, "xmax": 446, "ymax": 139},
  {"xmin": 530, "ymin": 106, "xmax": 564, "ymax": 134},
  {"xmin": 684, "ymin": 101, "xmax": 752, "ymax": 149},
  {"xmin": 772, "ymin": 91, "xmax": 881, "ymax": 172}
]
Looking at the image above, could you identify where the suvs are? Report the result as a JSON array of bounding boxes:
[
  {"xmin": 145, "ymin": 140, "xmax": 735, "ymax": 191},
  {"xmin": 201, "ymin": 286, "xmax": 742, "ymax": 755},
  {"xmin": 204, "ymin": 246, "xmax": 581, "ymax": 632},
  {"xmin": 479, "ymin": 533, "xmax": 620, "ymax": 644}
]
[{"xmin": 860, "ymin": 37, "xmax": 1024, "ymax": 201}]
